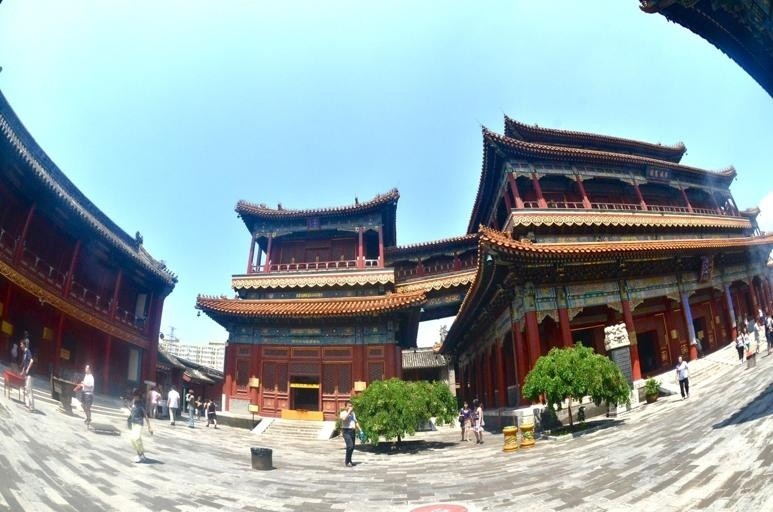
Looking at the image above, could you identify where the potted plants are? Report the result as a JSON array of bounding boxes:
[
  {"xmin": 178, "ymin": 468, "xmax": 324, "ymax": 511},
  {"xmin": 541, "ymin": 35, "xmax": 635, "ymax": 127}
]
[{"xmin": 643, "ymin": 375, "xmax": 661, "ymax": 403}]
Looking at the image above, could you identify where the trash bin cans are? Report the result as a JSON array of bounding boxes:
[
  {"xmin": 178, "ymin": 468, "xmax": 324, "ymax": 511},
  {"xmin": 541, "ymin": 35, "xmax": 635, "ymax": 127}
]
[{"xmin": 252, "ymin": 448, "xmax": 273, "ymax": 470}]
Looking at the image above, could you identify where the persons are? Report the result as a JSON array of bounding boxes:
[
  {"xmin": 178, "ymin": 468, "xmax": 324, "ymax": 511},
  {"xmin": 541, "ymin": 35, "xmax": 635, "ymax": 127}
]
[
  {"xmin": 147, "ymin": 385, "xmax": 217, "ymax": 430},
  {"xmin": 14, "ymin": 326, "xmax": 31, "ymax": 367},
  {"xmin": 121, "ymin": 392, "xmax": 153, "ymax": 463},
  {"xmin": 470, "ymin": 398, "xmax": 484, "ymax": 444},
  {"xmin": 735, "ymin": 312, "xmax": 773, "ymax": 367},
  {"xmin": 675, "ymin": 356, "xmax": 691, "ymax": 401},
  {"xmin": 458, "ymin": 400, "xmax": 471, "ymax": 441},
  {"xmin": 339, "ymin": 401, "xmax": 364, "ymax": 467},
  {"xmin": 72, "ymin": 363, "xmax": 94, "ymax": 425},
  {"xmin": 17, "ymin": 339, "xmax": 36, "ymax": 414}
]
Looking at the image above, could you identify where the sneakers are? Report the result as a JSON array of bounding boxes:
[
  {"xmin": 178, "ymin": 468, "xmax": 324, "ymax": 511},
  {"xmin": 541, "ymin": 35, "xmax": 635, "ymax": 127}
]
[{"xmin": 133, "ymin": 455, "xmax": 145, "ymax": 463}]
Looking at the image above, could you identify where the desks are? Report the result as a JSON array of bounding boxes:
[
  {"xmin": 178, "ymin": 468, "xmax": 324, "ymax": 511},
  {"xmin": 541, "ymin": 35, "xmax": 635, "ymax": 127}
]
[{"xmin": 3, "ymin": 369, "xmax": 25, "ymax": 404}]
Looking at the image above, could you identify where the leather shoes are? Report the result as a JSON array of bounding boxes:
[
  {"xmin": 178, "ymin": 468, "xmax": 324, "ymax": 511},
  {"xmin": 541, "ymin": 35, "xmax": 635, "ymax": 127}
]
[{"xmin": 347, "ymin": 462, "xmax": 353, "ymax": 467}]
